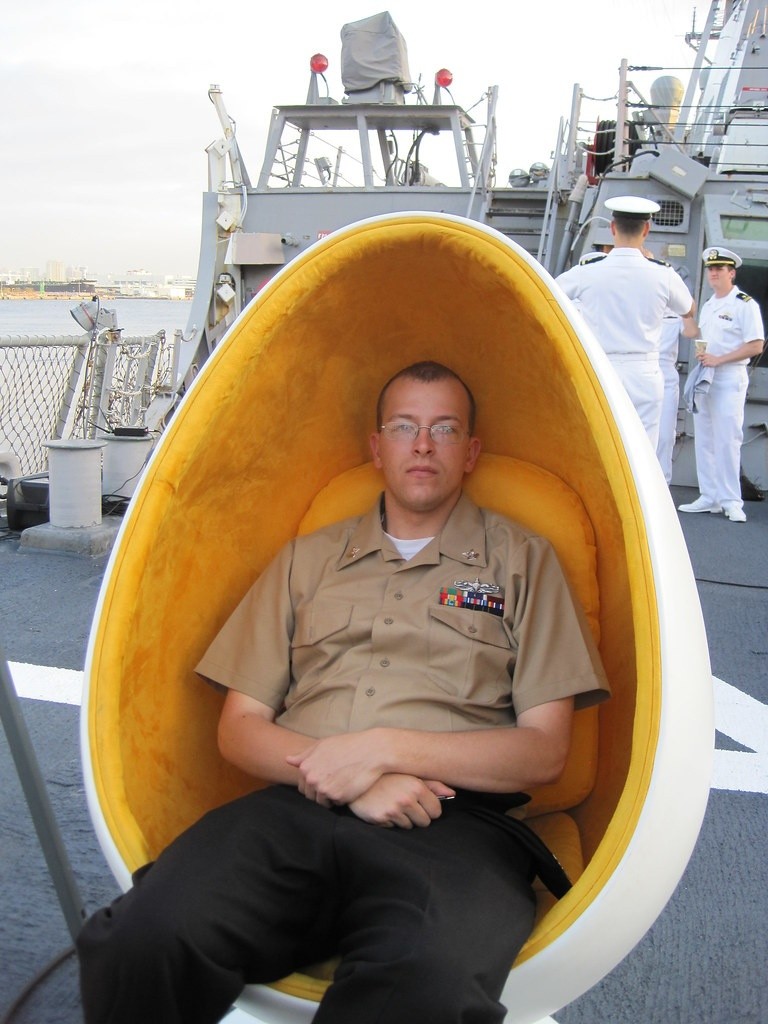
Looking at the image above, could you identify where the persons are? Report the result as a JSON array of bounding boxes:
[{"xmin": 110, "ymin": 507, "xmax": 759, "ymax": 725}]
[
  {"xmin": 678, "ymin": 247, "xmax": 765, "ymax": 523},
  {"xmin": 655, "ymin": 304, "xmax": 699, "ymax": 484},
  {"xmin": 555, "ymin": 195, "xmax": 696, "ymax": 453},
  {"xmin": 78, "ymin": 360, "xmax": 614, "ymax": 1024}
]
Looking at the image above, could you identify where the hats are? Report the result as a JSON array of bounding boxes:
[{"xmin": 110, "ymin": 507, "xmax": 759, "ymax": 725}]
[
  {"xmin": 604, "ymin": 196, "xmax": 662, "ymax": 220},
  {"xmin": 702, "ymin": 248, "xmax": 743, "ymax": 270},
  {"xmin": 579, "ymin": 252, "xmax": 608, "ymax": 265}
]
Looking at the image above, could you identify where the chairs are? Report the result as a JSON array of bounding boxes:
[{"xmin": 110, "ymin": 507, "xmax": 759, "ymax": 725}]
[{"xmin": 75, "ymin": 213, "xmax": 716, "ymax": 1024}]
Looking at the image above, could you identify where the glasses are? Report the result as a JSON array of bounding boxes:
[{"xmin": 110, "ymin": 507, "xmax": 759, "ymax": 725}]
[{"xmin": 377, "ymin": 422, "xmax": 470, "ymax": 444}]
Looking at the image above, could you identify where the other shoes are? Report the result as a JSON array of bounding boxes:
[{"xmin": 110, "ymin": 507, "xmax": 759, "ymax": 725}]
[
  {"xmin": 725, "ymin": 505, "xmax": 747, "ymax": 522},
  {"xmin": 678, "ymin": 498, "xmax": 723, "ymax": 513}
]
